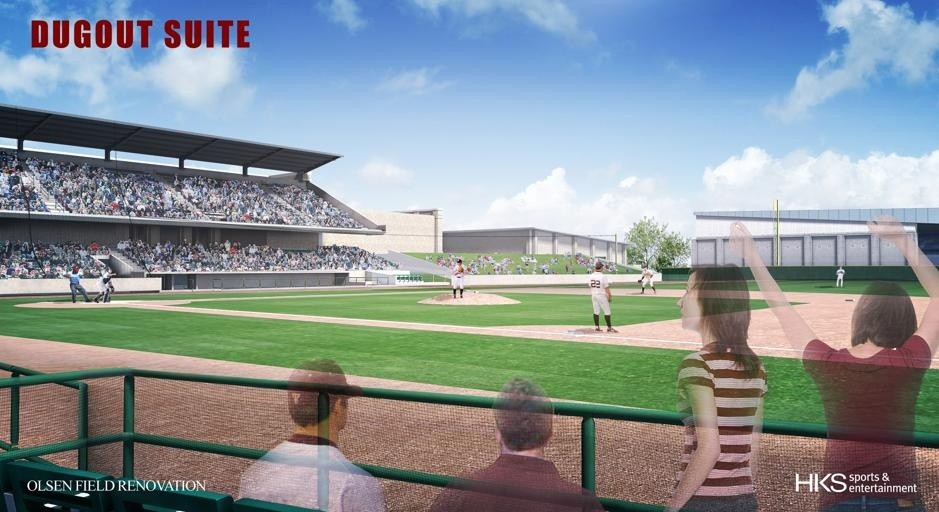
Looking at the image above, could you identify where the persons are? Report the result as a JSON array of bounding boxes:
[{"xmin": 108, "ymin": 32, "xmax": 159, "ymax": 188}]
[
  {"xmin": 432, "ymin": 375, "xmax": 605, "ymax": 510},
  {"xmin": 102, "ymin": 267, "xmax": 111, "ymax": 302},
  {"xmin": 451, "ymin": 258, "xmax": 468, "ymax": 300},
  {"xmin": 424, "ymin": 251, "xmax": 620, "ymax": 275},
  {"xmin": 834, "ymin": 265, "xmax": 847, "ymax": 288},
  {"xmin": 238, "ymin": 357, "xmax": 388, "ymax": 512},
  {"xmin": 727, "ymin": 213, "xmax": 939, "ymax": 510},
  {"xmin": 63, "ymin": 265, "xmax": 91, "ymax": 304},
  {"xmin": 0, "ymin": 147, "xmax": 400, "ymax": 281},
  {"xmin": 588, "ymin": 262, "xmax": 619, "ymax": 333},
  {"xmin": 93, "ymin": 266, "xmax": 117, "ymax": 303},
  {"xmin": 638, "ymin": 266, "xmax": 657, "ymax": 294},
  {"xmin": 667, "ymin": 262, "xmax": 770, "ymax": 510}
]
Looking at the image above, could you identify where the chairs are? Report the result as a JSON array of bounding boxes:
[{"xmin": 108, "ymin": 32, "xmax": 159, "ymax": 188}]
[{"xmin": 7, "ymin": 460, "xmax": 326, "ymax": 512}]
